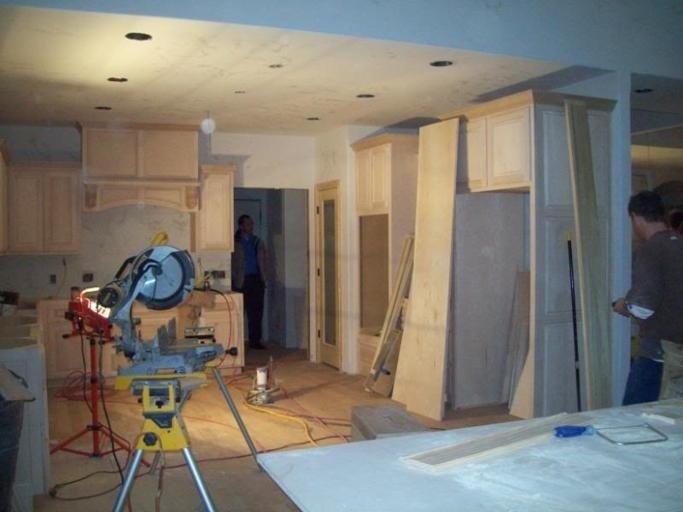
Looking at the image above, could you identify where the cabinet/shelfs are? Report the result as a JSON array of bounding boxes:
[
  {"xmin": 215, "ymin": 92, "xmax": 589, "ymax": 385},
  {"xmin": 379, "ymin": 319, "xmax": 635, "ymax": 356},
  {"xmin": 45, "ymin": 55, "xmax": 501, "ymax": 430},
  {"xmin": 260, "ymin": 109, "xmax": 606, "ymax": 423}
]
[
  {"xmin": 81, "ymin": 125, "xmax": 200, "ymax": 179},
  {"xmin": 180, "ymin": 294, "xmax": 244, "ymax": 376},
  {"xmin": 6, "ymin": 161, "xmax": 81, "ymax": 255},
  {"xmin": 357, "ymin": 142, "xmax": 393, "ymax": 379},
  {"xmin": 40, "ymin": 300, "xmax": 117, "ymax": 378},
  {"xmin": 103, "ymin": 301, "xmax": 179, "ymax": 379},
  {"xmin": 196, "ymin": 164, "xmax": 235, "ymax": 253},
  {"xmin": 457, "ymin": 103, "xmax": 532, "ymax": 193}
]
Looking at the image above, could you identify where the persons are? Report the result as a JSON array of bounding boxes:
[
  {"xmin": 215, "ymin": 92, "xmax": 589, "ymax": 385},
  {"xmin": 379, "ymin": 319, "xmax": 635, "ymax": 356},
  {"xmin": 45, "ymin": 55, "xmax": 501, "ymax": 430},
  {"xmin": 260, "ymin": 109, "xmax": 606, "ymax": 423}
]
[
  {"xmin": 232, "ymin": 215, "xmax": 267, "ymax": 350},
  {"xmin": 614, "ymin": 191, "xmax": 683, "ymax": 400}
]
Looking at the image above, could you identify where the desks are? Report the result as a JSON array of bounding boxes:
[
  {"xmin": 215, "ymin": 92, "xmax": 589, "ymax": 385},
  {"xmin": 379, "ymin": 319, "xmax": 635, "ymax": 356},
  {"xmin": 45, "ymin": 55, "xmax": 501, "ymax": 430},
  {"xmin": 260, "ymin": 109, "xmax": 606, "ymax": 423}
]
[{"xmin": 255, "ymin": 396, "xmax": 682, "ymax": 512}]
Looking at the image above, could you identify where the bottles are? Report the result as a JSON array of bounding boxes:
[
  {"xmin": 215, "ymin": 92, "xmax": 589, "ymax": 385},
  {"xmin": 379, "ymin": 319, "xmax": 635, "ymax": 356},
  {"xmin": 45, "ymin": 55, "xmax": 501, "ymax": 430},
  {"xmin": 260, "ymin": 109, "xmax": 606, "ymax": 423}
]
[{"xmin": 71, "ymin": 287, "xmax": 79, "ymax": 301}]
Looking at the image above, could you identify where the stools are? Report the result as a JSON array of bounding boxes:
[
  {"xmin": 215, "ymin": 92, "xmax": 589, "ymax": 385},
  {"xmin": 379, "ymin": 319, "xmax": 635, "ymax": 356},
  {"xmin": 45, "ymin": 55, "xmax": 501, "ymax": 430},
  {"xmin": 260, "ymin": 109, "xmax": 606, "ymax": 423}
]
[{"xmin": 351, "ymin": 403, "xmax": 425, "ymax": 441}]
